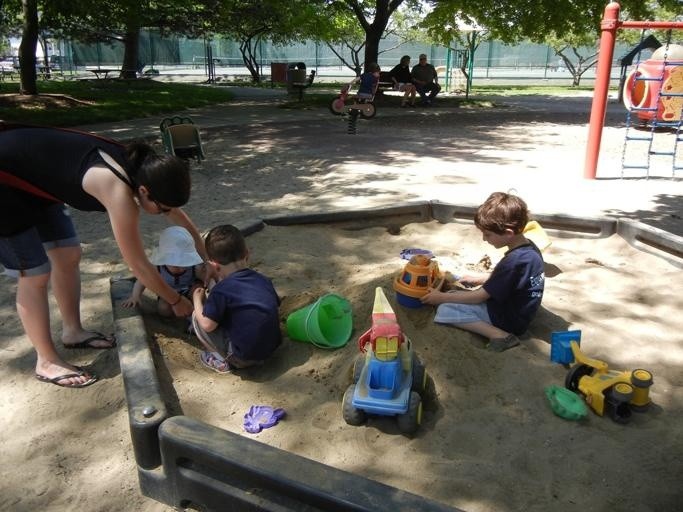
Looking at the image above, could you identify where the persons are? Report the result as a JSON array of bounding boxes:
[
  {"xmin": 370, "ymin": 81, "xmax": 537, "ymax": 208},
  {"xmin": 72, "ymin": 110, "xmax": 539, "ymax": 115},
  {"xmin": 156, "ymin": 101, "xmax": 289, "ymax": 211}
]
[
  {"xmin": 389, "ymin": 56, "xmax": 416, "ymax": 107},
  {"xmin": 410, "ymin": 53, "xmax": 440, "ymax": 104},
  {"xmin": 124, "ymin": 228, "xmax": 206, "ymax": 319},
  {"xmin": 189, "ymin": 224, "xmax": 283, "ymax": 373},
  {"xmin": 0, "ymin": 127, "xmax": 190, "ymax": 386},
  {"xmin": 352, "ymin": 63, "xmax": 380, "ymax": 96},
  {"xmin": 421, "ymin": 191, "xmax": 545, "ymax": 353}
]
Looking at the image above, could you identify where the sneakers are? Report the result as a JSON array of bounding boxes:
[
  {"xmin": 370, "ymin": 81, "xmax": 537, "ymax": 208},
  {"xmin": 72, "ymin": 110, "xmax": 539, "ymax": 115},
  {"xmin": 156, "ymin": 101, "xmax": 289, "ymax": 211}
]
[
  {"xmin": 199, "ymin": 351, "xmax": 236, "ymax": 374},
  {"xmin": 487, "ymin": 333, "xmax": 521, "ymax": 352}
]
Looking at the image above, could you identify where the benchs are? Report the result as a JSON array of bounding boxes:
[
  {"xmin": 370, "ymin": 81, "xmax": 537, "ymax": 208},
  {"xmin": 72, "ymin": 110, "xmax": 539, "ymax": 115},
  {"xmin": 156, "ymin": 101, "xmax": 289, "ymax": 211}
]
[
  {"xmin": 359, "ymin": 70, "xmax": 430, "ymax": 100},
  {"xmin": 0, "ymin": 51, "xmax": 148, "ymax": 87},
  {"xmin": 291, "ymin": 69, "xmax": 318, "ymax": 108}
]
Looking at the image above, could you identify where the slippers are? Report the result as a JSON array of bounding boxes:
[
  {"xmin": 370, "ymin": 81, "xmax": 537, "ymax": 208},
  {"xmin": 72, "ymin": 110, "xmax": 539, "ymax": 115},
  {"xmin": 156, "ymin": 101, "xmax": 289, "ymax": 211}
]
[
  {"xmin": 63, "ymin": 331, "xmax": 116, "ymax": 349},
  {"xmin": 35, "ymin": 365, "xmax": 99, "ymax": 388}
]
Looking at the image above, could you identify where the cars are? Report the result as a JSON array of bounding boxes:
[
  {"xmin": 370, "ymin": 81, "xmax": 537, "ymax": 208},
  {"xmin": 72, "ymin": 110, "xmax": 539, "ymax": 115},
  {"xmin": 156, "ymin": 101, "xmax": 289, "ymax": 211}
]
[{"xmin": 0, "ymin": 52, "xmax": 68, "ymax": 74}]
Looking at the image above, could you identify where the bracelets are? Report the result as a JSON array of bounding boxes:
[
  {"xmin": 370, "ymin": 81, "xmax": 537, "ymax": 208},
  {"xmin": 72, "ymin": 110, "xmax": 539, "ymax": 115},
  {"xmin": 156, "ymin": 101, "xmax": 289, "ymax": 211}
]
[{"xmin": 169, "ymin": 295, "xmax": 185, "ymax": 305}]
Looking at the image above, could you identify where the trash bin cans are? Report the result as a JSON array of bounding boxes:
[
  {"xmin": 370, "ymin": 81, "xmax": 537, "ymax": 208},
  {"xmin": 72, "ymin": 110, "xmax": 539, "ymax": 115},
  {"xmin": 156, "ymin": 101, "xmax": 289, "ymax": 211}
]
[{"xmin": 286, "ymin": 62, "xmax": 306, "ymax": 95}]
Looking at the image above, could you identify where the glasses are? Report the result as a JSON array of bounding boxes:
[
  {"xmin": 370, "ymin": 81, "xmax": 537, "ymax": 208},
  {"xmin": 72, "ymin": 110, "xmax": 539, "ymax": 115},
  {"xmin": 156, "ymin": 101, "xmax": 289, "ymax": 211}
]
[{"xmin": 150, "ymin": 198, "xmax": 171, "ymax": 213}]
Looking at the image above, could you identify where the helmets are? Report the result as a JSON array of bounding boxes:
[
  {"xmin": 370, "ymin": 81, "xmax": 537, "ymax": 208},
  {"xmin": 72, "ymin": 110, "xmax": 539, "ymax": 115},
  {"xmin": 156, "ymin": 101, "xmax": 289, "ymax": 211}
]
[{"xmin": 366, "ymin": 62, "xmax": 380, "ymax": 72}]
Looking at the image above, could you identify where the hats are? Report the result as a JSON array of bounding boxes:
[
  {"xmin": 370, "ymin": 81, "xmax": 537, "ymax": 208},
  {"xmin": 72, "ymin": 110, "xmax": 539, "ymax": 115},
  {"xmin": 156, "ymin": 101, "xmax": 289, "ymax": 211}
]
[{"xmin": 150, "ymin": 225, "xmax": 204, "ymax": 268}]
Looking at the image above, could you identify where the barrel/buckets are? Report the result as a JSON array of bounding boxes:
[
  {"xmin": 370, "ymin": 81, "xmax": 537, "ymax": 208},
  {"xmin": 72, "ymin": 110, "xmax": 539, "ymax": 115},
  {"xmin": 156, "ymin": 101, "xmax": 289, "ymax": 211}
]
[{"xmin": 287, "ymin": 293, "xmax": 353, "ymax": 350}]
